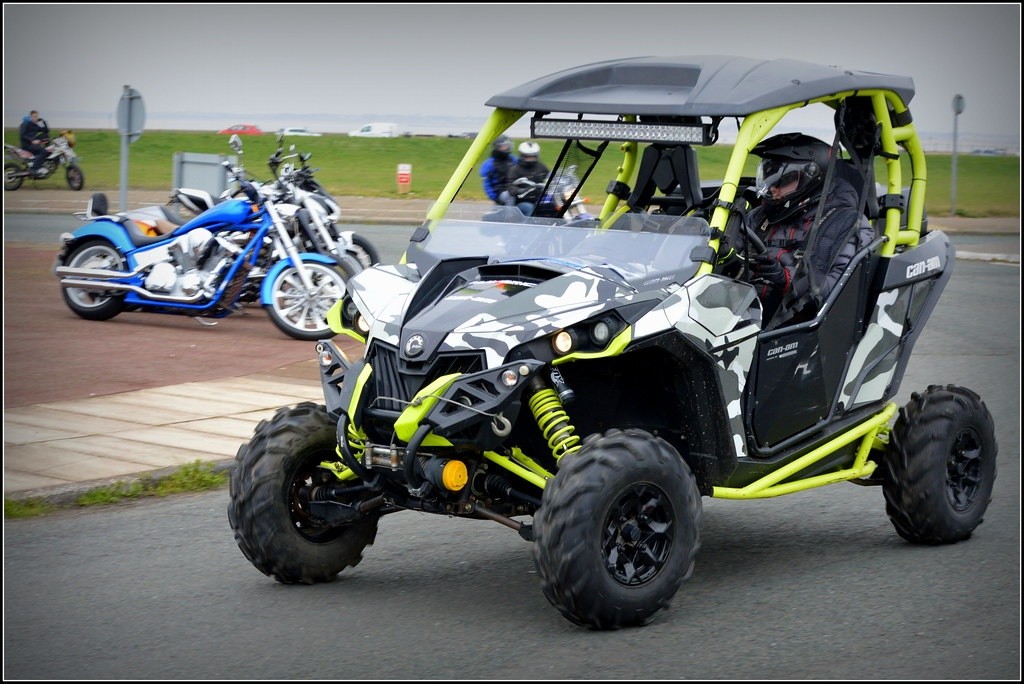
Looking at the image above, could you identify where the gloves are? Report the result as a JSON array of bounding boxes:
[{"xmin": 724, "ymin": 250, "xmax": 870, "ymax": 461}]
[
  {"xmin": 754, "ymin": 258, "xmax": 791, "ymax": 293},
  {"xmin": 495, "ymin": 198, "xmax": 505, "ymax": 205},
  {"xmin": 509, "ymin": 186, "xmax": 519, "ymax": 196}
]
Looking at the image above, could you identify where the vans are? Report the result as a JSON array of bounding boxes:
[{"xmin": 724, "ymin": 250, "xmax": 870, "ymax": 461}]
[{"xmin": 348, "ymin": 122, "xmax": 397, "ymax": 138}]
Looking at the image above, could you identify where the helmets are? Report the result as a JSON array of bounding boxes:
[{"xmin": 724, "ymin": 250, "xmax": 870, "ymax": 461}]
[
  {"xmin": 518, "ymin": 141, "xmax": 540, "ymax": 156},
  {"xmin": 749, "ymin": 132, "xmax": 838, "ymax": 225},
  {"xmin": 493, "ymin": 134, "xmax": 511, "ymax": 148}
]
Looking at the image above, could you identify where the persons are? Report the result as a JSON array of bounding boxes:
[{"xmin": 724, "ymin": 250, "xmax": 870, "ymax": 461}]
[
  {"xmin": 18, "ymin": 110, "xmax": 50, "ymax": 177},
  {"xmin": 480, "ymin": 135, "xmax": 550, "ymax": 217},
  {"xmin": 736, "ymin": 133, "xmax": 873, "ymax": 334}
]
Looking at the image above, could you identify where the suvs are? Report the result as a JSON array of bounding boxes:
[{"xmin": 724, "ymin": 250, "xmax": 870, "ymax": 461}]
[{"xmin": 228, "ymin": 52, "xmax": 999, "ymax": 631}]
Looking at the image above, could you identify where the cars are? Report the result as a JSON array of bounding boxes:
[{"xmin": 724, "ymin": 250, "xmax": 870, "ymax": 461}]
[
  {"xmin": 275, "ymin": 127, "xmax": 322, "ymax": 137},
  {"xmin": 217, "ymin": 125, "xmax": 261, "ymax": 136}
]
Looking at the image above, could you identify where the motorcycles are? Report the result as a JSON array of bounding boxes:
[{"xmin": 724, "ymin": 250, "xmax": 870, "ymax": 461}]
[
  {"xmin": 4, "ymin": 128, "xmax": 84, "ymax": 191},
  {"xmin": 481, "ymin": 164, "xmax": 599, "ymax": 256},
  {"xmin": 52, "ymin": 131, "xmax": 377, "ymax": 342}
]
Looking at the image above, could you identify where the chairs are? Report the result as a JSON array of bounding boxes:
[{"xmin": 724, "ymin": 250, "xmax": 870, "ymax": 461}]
[{"xmin": 622, "ymin": 145, "xmax": 720, "ymax": 268}]
